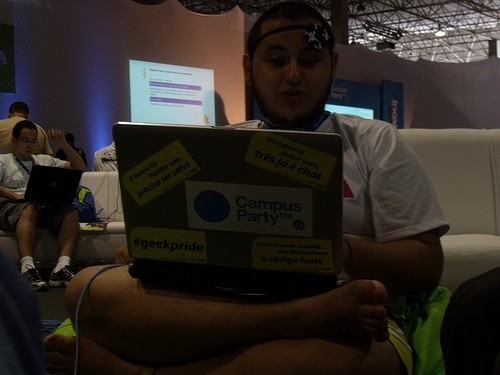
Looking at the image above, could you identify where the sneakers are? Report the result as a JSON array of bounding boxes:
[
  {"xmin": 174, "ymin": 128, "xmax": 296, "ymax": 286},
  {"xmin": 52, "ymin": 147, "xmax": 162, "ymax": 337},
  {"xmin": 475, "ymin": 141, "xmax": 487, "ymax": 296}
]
[
  {"xmin": 49, "ymin": 265, "xmax": 77, "ymax": 286},
  {"xmin": 23, "ymin": 264, "xmax": 48, "ymax": 291}
]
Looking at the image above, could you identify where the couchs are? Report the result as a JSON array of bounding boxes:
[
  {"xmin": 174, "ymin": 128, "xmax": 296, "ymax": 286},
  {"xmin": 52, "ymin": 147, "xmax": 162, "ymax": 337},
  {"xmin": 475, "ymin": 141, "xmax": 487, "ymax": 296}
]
[
  {"xmin": 0, "ymin": 170, "xmax": 128, "ymax": 263},
  {"xmin": 394, "ymin": 127, "xmax": 500, "ymax": 295}
]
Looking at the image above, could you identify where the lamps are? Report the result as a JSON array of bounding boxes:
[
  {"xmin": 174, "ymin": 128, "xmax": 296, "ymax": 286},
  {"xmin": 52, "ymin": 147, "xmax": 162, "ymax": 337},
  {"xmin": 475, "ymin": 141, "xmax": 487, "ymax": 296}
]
[
  {"xmin": 376, "ymin": 39, "xmax": 395, "ymax": 51},
  {"xmin": 435, "ymin": 24, "xmax": 447, "ymax": 37}
]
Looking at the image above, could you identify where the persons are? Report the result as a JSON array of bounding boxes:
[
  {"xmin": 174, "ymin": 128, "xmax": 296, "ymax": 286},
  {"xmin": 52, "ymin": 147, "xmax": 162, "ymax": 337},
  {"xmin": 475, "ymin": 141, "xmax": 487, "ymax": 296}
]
[
  {"xmin": 56, "ymin": 133, "xmax": 88, "ymax": 166},
  {"xmin": 0, "ymin": 249, "xmax": 44, "ymax": 375},
  {"xmin": 0, "ymin": 101, "xmax": 52, "ymax": 156},
  {"xmin": 0, "ymin": 121, "xmax": 79, "ymax": 292},
  {"xmin": 93, "ymin": 140, "xmax": 119, "ymax": 172},
  {"xmin": 43, "ymin": 2, "xmax": 450, "ymax": 375}
]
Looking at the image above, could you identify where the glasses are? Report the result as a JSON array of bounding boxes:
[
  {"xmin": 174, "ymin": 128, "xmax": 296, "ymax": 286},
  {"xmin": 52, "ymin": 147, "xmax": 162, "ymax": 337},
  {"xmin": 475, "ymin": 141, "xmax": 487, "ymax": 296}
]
[{"xmin": 17, "ymin": 138, "xmax": 38, "ymax": 145}]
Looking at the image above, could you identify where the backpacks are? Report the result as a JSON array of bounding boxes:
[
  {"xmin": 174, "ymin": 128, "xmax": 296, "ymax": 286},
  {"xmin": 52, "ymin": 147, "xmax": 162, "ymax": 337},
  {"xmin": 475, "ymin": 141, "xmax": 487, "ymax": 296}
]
[{"xmin": 72, "ymin": 185, "xmax": 97, "ymax": 222}]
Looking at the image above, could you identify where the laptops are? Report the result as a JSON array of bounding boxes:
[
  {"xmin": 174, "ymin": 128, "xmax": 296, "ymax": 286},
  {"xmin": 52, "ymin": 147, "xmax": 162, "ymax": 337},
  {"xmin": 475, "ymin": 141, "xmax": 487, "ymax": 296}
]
[
  {"xmin": 10, "ymin": 165, "xmax": 83, "ymax": 206},
  {"xmin": 113, "ymin": 122, "xmax": 350, "ymax": 302}
]
